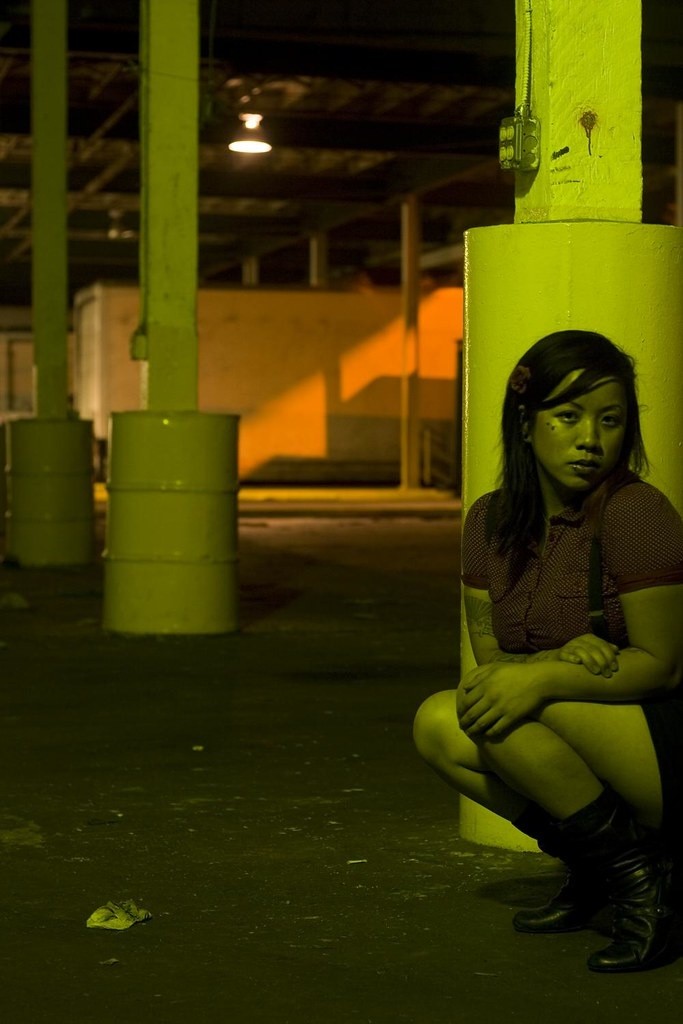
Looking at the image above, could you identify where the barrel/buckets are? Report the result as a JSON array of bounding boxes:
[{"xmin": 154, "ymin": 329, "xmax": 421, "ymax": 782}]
[
  {"xmin": 6, "ymin": 418, "xmax": 96, "ymax": 567},
  {"xmin": 101, "ymin": 412, "xmax": 241, "ymax": 635}
]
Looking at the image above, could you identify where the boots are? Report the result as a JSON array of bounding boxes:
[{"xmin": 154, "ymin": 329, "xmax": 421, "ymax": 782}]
[
  {"xmin": 560, "ymin": 777, "xmax": 683, "ymax": 974},
  {"xmin": 511, "ymin": 796, "xmax": 616, "ymax": 934}
]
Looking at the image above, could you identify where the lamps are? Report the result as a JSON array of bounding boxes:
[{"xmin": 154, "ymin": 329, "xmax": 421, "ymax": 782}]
[{"xmin": 227, "ymin": 119, "xmax": 274, "ymax": 152}]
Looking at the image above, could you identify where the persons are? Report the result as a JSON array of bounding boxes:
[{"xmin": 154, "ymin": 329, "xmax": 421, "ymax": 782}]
[{"xmin": 414, "ymin": 327, "xmax": 683, "ymax": 970}]
